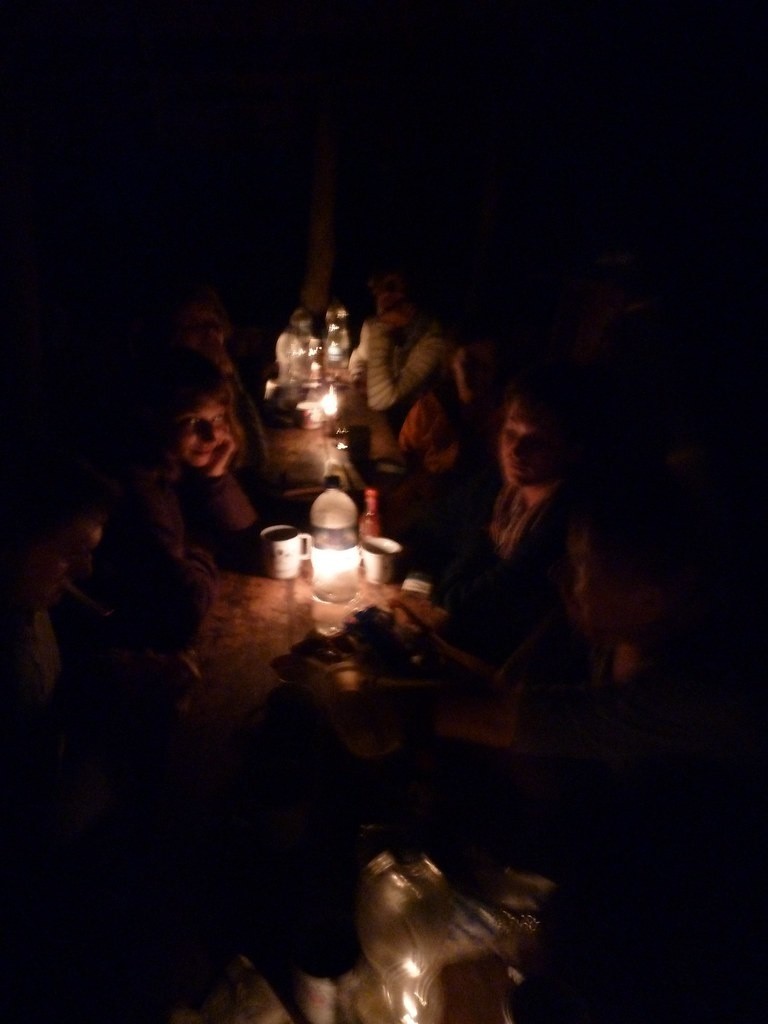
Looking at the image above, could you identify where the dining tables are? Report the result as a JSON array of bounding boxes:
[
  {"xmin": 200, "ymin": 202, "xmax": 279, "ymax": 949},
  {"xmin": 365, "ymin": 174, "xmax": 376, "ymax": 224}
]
[
  {"xmin": 185, "ymin": 568, "xmax": 444, "ymax": 756},
  {"xmin": 259, "ymin": 363, "xmax": 409, "ymax": 499}
]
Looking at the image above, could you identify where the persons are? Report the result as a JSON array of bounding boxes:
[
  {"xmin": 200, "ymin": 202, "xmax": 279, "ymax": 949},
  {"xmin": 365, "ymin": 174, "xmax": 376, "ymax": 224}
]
[
  {"xmin": 419, "ymin": 362, "xmax": 598, "ymax": 644},
  {"xmin": 386, "ymin": 495, "xmax": 744, "ymax": 908},
  {"xmin": 150, "ymin": 282, "xmax": 270, "ymax": 474},
  {"xmin": 100, "ymin": 358, "xmax": 259, "ymax": 632},
  {"xmin": 396, "ymin": 335, "xmax": 522, "ymax": 539},
  {"xmin": 0, "ymin": 458, "xmax": 206, "ymax": 830},
  {"xmin": 349, "ymin": 258, "xmax": 457, "ymax": 436}
]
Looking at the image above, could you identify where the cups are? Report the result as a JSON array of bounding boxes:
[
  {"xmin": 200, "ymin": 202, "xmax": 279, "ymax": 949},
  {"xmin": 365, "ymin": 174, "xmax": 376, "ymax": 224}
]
[
  {"xmin": 295, "ymin": 400, "xmax": 322, "ymax": 429},
  {"xmin": 363, "ymin": 536, "xmax": 403, "ymax": 585},
  {"xmin": 260, "ymin": 524, "xmax": 312, "ymax": 580}
]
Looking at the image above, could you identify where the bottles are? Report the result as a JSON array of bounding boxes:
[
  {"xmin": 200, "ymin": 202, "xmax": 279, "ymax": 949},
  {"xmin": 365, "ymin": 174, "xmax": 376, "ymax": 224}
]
[
  {"xmin": 358, "ymin": 487, "xmax": 382, "ymax": 540},
  {"xmin": 347, "ymin": 851, "xmax": 449, "ymax": 1024},
  {"xmin": 310, "ymin": 475, "xmax": 362, "ymax": 604}
]
